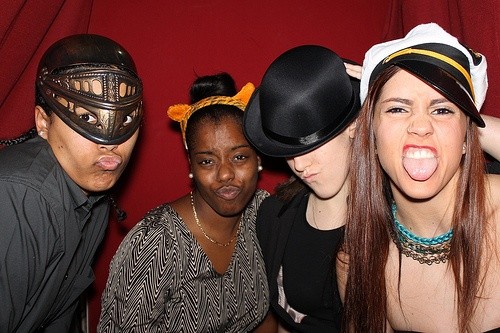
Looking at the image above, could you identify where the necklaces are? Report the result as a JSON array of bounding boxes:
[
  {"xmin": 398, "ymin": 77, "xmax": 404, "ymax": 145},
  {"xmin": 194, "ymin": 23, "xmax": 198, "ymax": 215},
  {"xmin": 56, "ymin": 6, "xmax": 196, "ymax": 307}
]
[
  {"xmin": 190, "ymin": 191, "xmax": 243, "ymax": 247},
  {"xmin": 312, "ymin": 195, "xmax": 348, "ymax": 231},
  {"xmin": 387, "ymin": 203, "xmax": 466, "ymax": 267}
]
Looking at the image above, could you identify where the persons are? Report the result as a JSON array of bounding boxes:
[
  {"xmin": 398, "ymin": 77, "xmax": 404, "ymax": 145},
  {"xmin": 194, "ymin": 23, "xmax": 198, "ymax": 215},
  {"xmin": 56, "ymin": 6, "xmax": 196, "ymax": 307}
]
[
  {"xmin": 244, "ymin": 45, "xmax": 499, "ymax": 333},
  {"xmin": 337, "ymin": 23, "xmax": 500, "ymax": 333},
  {"xmin": 1, "ymin": 34, "xmax": 142, "ymax": 333},
  {"xmin": 97, "ymin": 75, "xmax": 279, "ymax": 333}
]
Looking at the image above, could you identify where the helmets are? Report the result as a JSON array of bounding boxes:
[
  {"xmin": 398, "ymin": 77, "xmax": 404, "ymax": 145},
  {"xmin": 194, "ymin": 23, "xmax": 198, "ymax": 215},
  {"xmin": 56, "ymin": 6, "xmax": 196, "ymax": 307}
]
[{"xmin": 36, "ymin": 33, "xmax": 143, "ymax": 145}]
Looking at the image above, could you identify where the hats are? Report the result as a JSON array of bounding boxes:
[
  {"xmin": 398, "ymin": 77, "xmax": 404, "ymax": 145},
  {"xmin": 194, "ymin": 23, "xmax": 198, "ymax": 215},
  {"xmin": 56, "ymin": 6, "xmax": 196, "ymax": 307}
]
[
  {"xmin": 242, "ymin": 45, "xmax": 363, "ymax": 158},
  {"xmin": 359, "ymin": 22, "xmax": 489, "ymax": 128}
]
